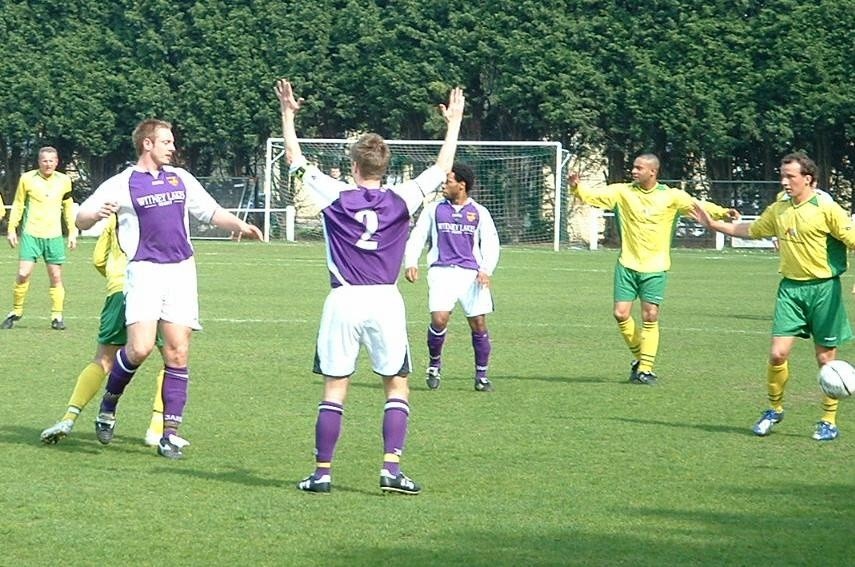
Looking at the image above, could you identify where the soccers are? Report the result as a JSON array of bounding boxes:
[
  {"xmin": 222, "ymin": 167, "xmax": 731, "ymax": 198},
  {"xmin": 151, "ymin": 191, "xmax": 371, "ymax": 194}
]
[{"xmin": 819, "ymin": 359, "xmax": 855, "ymax": 399}]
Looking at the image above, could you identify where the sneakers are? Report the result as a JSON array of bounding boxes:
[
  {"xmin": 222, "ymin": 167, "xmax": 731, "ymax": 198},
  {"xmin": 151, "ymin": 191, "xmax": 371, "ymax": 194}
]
[
  {"xmin": 95, "ymin": 409, "xmax": 116, "ymax": 445},
  {"xmin": 158, "ymin": 438, "xmax": 185, "ymax": 459},
  {"xmin": 475, "ymin": 376, "xmax": 495, "ymax": 391},
  {"xmin": 380, "ymin": 469, "xmax": 421, "ymax": 495},
  {"xmin": 1, "ymin": 312, "xmax": 24, "ymax": 329},
  {"xmin": 629, "ymin": 360, "xmax": 658, "ymax": 386},
  {"xmin": 296, "ymin": 473, "xmax": 331, "ymax": 495},
  {"xmin": 51, "ymin": 319, "xmax": 66, "ymax": 330},
  {"xmin": 425, "ymin": 367, "xmax": 441, "ymax": 390},
  {"xmin": 145, "ymin": 429, "xmax": 190, "ymax": 447},
  {"xmin": 753, "ymin": 408, "xmax": 784, "ymax": 437},
  {"xmin": 40, "ymin": 419, "xmax": 74, "ymax": 445},
  {"xmin": 811, "ymin": 421, "xmax": 838, "ymax": 441}
]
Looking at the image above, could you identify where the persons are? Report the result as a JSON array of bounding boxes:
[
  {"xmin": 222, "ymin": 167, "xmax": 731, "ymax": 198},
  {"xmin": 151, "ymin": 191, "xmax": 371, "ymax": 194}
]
[
  {"xmin": 686, "ymin": 146, "xmax": 855, "ymax": 441},
  {"xmin": 404, "ymin": 164, "xmax": 500, "ymax": 392},
  {"xmin": 565, "ymin": 154, "xmax": 741, "ymax": 386},
  {"xmin": 328, "ymin": 167, "xmax": 348, "ymax": 185},
  {"xmin": 75, "ymin": 118, "xmax": 265, "ymax": 462},
  {"xmin": 39, "ymin": 212, "xmax": 190, "ymax": 447},
  {"xmin": 0, "ymin": 147, "xmax": 78, "ymax": 331},
  {"xmin": 270, "ymin": 77, "xmax": 465, "ymax": 497}
]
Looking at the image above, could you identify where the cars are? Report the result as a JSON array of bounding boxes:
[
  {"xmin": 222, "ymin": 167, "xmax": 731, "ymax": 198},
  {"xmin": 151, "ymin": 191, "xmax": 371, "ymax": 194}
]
[{"xmin": 677, "ymin": 216, "xmax": 708, "ymax": 237}]
